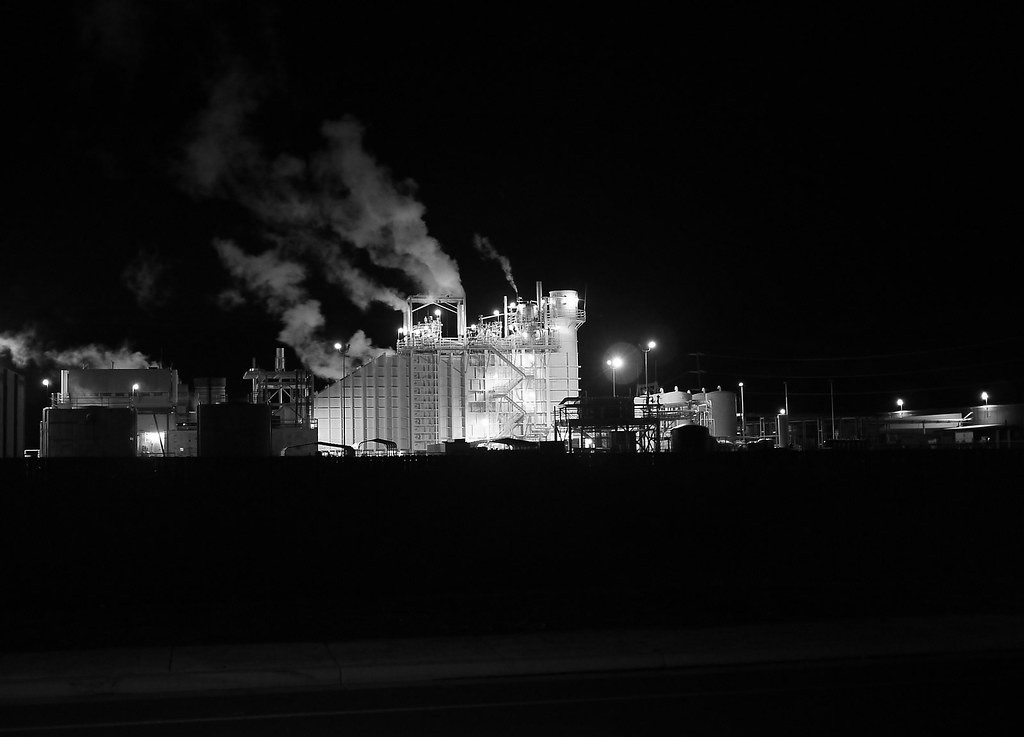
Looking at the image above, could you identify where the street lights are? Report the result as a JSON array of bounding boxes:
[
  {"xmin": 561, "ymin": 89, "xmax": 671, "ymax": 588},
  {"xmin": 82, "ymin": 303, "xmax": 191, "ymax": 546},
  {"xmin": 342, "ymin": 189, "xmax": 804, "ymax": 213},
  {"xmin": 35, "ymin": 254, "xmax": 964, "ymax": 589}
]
[
  {"xmin": 642, "ymin": 340, "xmax": 655, "ymax": 403},
  {"xmin": 607, "ymin": 359, "xmax": 621, "ymax": 397},
  {"xmin": 738, "ymin": 382, "xmax": 746, "ymax": 443},
  {"xmin": 897, "ymin": 399, "xmax": 903, "ymax": 411},
  {"xmin": 981, "ymin": 392, "xmax": 989, "ymax": 405},
  {"xmin": 333, "ymin": 341, "xmax": 351, "ymax": 457}
]
